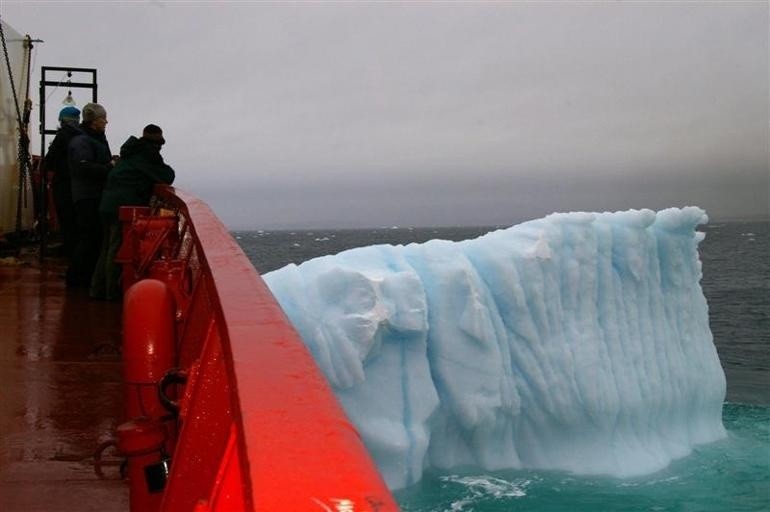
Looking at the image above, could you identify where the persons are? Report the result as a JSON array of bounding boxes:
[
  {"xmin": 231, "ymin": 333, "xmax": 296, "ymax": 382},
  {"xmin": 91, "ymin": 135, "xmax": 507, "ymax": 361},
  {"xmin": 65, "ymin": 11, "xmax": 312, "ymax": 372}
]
[{"xmin": 39, "ymin": 104, "xmax": 176, "ymax": 303}]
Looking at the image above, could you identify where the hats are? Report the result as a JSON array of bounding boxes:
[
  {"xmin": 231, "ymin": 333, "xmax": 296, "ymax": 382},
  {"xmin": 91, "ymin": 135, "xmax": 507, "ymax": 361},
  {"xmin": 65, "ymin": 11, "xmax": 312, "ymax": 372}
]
[
  {"xmin": 142, "ymin": 123, "xmax": 166, "ymax": 144},
  {"xmin": 59, "ymin": 106, "xmax": 81, "ymax": 125},
  {"xmin": 82, "ymin": 102, "xmax": 107, "ymax": 118}
]
